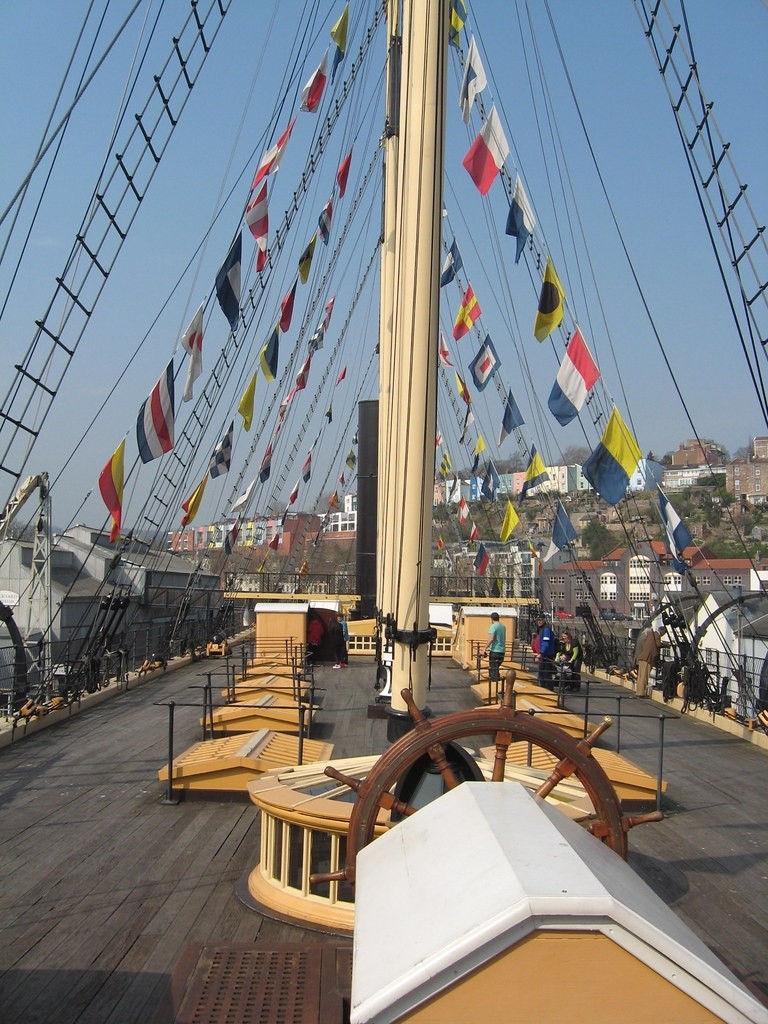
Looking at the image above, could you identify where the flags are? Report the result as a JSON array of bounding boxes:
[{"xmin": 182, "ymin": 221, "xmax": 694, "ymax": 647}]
[
  {"xmin": 432, "ymin": 1, "xmax": 697, "ymax": 574},
  {"xmin": 98, "ymin": 1, "xmax": 359, "ymax": 576}
]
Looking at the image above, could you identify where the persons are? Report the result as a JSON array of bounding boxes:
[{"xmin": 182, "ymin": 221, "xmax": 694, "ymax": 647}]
[
  {"xmin": 533, "ymin": 613, "xmax": 582, "ymax": 691},
  {"xmin": 483, "ymin": 612, "xmax": 506, "ymax": 680},
  {"xmin": 308, "ymin": 615, "xmax": 348, "ymax": 668},
  {"xmin": 634, "ymin": 626, "xmax": 668, "ymax": 698}
]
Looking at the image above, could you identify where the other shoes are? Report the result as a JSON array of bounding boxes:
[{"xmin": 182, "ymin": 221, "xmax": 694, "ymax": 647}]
[
  {"xmin": 636, "ymin": 695, "xmax": 649, "ymax": 699},
  {"xmin": 572, "ymin": 687, "xmax": 578, "ymax": 692},
  {"xmin": 565, "ymin": 685, "xmax": 572, "ymax": 690},
  {"xmin": 333, "ymin": 661, "xmax": 349, "ymax": 668}
]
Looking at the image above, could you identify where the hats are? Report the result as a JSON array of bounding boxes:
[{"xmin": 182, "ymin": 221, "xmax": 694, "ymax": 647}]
[{"xmin": 536, "ymin": 613, "xmax": 546, "ymax": 619}]
